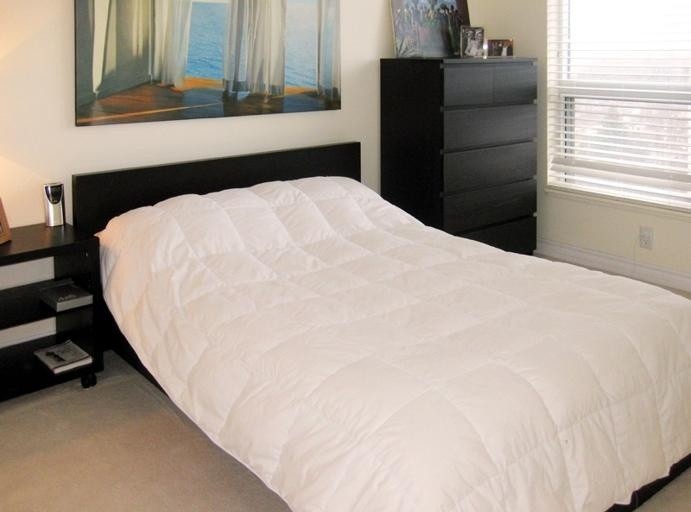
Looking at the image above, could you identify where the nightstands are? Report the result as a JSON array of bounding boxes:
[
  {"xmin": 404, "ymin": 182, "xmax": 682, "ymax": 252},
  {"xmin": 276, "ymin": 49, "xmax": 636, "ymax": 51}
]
[{"xmin": 1, "ymin": 220, "xmax": 108, "ymax": 408}]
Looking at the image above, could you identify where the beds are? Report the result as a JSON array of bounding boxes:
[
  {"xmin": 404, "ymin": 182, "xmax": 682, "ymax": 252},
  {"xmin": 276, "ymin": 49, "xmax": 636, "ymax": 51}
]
[{"xmin": 78, "ymin": 147, "xmax": 691, "ymax": 512}]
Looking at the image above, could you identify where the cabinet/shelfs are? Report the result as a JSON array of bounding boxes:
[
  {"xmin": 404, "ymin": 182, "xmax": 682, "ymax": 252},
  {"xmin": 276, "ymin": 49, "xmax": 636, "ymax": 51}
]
[{"xmin": 379, "ymin": 51, "xmax": 542, "ymax": 258}]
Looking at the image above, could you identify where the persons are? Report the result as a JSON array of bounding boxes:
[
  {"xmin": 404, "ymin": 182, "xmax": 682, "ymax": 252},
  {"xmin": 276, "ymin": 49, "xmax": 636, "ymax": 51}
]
[
  {"xmin": 488, "ymin": 39, "xmax": 513, "ymax": 57},
  {"xmin": 394, "ymin": 4, "xmax": 484, "ymax": 57}
]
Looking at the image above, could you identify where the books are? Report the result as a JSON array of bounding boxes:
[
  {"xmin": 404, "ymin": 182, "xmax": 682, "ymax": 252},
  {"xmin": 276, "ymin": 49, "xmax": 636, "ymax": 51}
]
[
  {"xmin": 35, "ymin": 339, "xmax": 94, "ymax": 374},
  {"xmin": 35, "ymin": 278, "xmax": 94, "ymax": 312}
]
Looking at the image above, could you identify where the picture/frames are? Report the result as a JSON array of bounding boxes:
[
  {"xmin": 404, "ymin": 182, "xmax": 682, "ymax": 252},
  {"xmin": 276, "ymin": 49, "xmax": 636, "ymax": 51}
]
[{"xmin": 458, "ymin": 25, "xmax": 516, "ymax": 59}]
